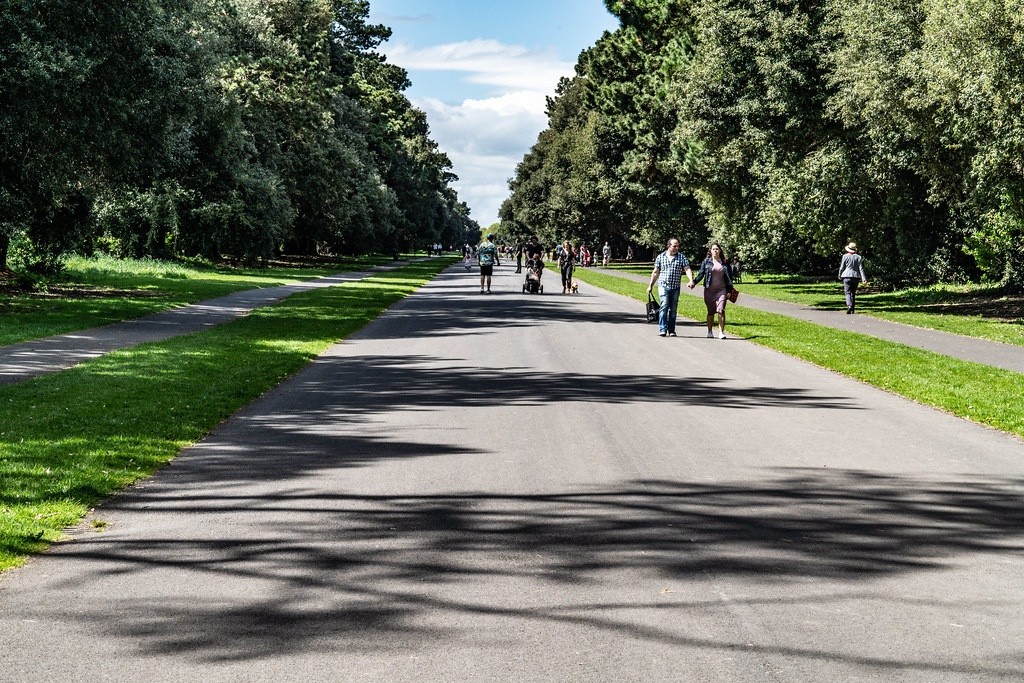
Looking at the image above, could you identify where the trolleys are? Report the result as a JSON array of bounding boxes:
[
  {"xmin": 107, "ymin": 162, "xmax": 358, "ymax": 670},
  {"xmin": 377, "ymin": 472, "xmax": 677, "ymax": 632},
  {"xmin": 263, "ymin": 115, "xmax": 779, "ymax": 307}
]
[{"xmin": 523, "ymin": 259, "xmax": 544, "ymax": 296}]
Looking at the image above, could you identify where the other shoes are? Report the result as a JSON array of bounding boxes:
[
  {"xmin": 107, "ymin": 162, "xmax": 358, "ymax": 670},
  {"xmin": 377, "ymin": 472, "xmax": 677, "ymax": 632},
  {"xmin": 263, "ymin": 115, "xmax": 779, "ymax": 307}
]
[
  {"xmin": 719, "ymin": 332, "xmax": 726, "ymax": 339},
  {"xmin": 706, "ymin": 332, "xmax": 714, "ymax": 338},
  {"xmin": 515, "ymin": 270, "xmax": 521, "ymax": 274},
  {"xmin": 668, "ymin": 331, "xmax": 676, "ymax": 337},
  {"xmin": 487, "ymin": 290, "xmax": 491, "ymax": 294},
  {"xmin": 480, "ymin": 290, "xmax": 484, "ymax": 294},
  {"xmin": 568, "ymin": 288, "xmax": 571, "ymax": 294},
  {"xmin": 562, "ymin": 288, "xmax": 566, "ymax": 296},
  {"xmin": 657, "ymin": 332, "xmax": 666, "ymax": 337}
]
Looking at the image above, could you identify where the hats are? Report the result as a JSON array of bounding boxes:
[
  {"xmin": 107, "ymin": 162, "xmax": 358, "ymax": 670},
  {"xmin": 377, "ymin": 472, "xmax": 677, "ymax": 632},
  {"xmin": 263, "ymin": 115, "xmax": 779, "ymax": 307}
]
[
  {"xmin": 486, "ymin": 233, "xmax": 494, "ymax": 239},
  {"xmin": 844, "ymin": 242, "xmax": 857, "ymax": 254}
]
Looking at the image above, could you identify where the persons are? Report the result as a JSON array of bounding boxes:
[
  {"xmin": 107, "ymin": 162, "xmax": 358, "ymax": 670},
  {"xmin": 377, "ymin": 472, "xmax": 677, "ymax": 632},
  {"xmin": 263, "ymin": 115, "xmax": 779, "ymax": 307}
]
[
  {"xmin": 626, "ymin": 246, "xmax": 633, "ymax": 263},
  {"xmin": 461, "ymin": 243, "xmax": 480, "ymax": 272},
  {"xmin": 478, "ymin": 233, "xmax": 578, "ymax": 294},
  {"xmin": 580, "ymin": 241, "xmax": 611, "ymax": 267},
  {"xmin": 838, "ymin": 242, "xmax": 867, "ymax": 314},
  {"xmin": 646, "ymin": 238, "xmax": 741, "ymax": 339},
  {"xmin": 427, "ymin": 242, "xmax": 459, "ymax": 258}
]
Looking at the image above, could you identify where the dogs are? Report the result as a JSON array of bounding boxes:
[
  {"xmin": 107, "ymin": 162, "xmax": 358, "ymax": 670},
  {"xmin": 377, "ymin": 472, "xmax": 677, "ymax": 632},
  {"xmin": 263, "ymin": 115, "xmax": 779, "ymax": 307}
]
[{"xmin": 572, "ymin": 282, "xmax": 578, "ymax": 293}]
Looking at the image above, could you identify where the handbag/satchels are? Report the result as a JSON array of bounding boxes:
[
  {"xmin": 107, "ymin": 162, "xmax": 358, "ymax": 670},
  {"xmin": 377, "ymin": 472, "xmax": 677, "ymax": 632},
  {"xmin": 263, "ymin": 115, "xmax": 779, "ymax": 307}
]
[
  {"xmin": 645, "ymin": 292, "xmax": 660, "ymax": 323},
  {"xmin": 728, "ymin": 288, "xmax": 739, "ymax": 303}
]
